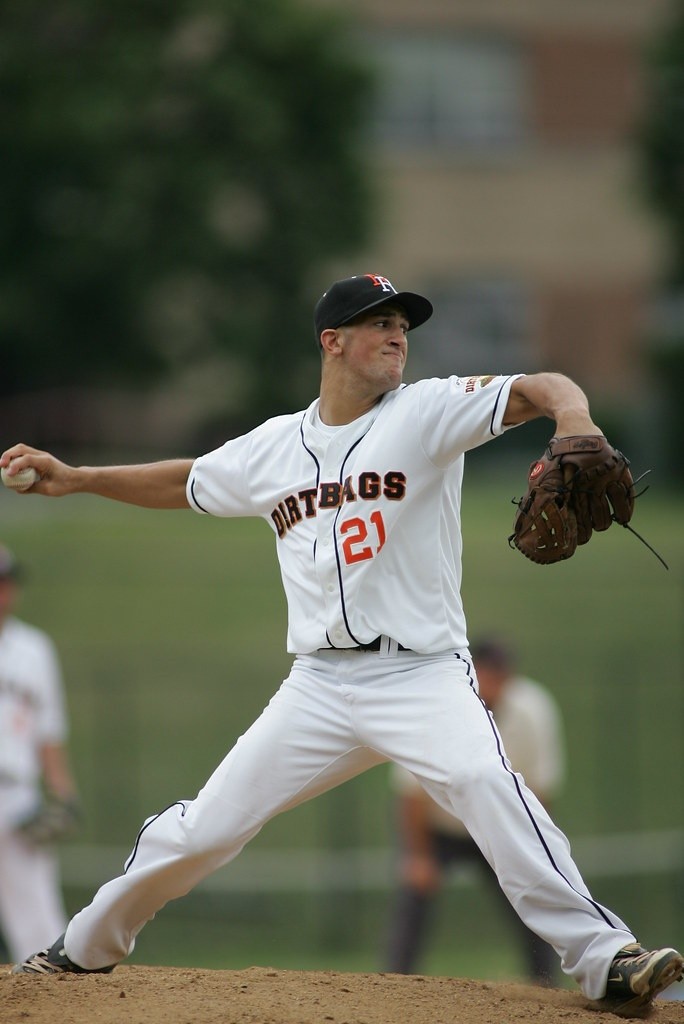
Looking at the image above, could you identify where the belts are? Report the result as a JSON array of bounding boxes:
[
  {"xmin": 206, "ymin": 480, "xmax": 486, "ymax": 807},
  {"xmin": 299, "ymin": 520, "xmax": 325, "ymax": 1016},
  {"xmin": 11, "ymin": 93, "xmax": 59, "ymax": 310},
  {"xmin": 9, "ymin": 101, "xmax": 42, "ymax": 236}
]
[{"xmin": 319, "ymin": 634, "xmax": 410, "ymax": 651}]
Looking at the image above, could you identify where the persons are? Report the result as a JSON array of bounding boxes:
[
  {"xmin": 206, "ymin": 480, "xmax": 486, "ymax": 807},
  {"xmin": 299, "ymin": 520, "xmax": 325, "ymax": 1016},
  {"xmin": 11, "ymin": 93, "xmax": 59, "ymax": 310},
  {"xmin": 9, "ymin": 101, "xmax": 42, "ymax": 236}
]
[
  {"xmin": 0, "ymin": 275, "xmax": 681, "ymax": 1019},
  {"xmin": 0, "ymin": 547, "xmax": 79, "ymax": 968},
  {"xmin": 395, "ymin": 628, "xmax": 571, "ymax": 984}
]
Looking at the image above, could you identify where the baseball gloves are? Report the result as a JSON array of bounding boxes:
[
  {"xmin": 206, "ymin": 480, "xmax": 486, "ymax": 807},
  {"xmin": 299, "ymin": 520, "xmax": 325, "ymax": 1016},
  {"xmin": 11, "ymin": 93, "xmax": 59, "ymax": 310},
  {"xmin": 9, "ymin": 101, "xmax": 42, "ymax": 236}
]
[
  {"xmin": 505, "ymin": 430, "xmax": 636, "ymax": 567},
  {"xmin": 15, "ymin": 797, "xmax": 81, "ymax": 850}
]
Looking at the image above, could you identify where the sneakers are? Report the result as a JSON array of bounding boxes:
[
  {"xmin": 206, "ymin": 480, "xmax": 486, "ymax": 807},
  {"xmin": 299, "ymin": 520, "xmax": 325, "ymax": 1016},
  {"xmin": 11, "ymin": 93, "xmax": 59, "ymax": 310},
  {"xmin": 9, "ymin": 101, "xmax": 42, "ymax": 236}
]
[
  {"xmin": 9, "ymin": 932, "xmax": 117, "ymax": 978},
  {"xmin": 597, "ymin": 942, "xmax": 684, "ymax": 1015}
]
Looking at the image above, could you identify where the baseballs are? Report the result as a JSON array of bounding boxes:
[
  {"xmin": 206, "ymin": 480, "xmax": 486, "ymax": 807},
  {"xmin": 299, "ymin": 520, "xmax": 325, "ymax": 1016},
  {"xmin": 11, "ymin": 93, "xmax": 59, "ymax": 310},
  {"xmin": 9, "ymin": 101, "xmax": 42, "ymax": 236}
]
[{"xmin": 1, "ymin": 458, "xmax": 36, "ymax": 493}]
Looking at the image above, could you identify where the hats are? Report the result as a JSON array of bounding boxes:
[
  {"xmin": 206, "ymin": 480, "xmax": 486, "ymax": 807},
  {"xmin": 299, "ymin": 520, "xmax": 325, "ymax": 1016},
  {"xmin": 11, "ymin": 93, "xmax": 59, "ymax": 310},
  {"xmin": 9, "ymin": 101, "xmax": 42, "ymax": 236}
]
[
  {"xmin": 313, "ymin": 273, "xmax": 433, "ymax": 349},
  {"xmin": 1, "ymin": 546, "xmax": 23, "ymax": 580}
]
